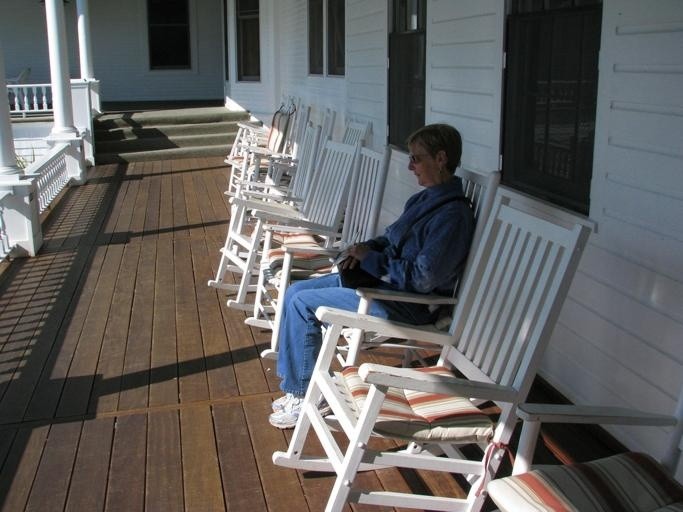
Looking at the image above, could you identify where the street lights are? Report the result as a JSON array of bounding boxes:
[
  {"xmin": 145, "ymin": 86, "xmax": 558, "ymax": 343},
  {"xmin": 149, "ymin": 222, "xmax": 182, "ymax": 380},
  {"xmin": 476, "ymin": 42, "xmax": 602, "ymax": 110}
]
[{"xmin": 336, "ymin": 240, "xmax": 384, "ymax": 287}]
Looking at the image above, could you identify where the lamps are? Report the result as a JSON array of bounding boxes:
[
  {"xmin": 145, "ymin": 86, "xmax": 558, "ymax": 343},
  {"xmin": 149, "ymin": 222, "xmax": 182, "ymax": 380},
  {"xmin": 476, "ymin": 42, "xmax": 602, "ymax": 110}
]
[{"xmin": 6, "ymin": 66, "xmax": 31, "ymax": 109}]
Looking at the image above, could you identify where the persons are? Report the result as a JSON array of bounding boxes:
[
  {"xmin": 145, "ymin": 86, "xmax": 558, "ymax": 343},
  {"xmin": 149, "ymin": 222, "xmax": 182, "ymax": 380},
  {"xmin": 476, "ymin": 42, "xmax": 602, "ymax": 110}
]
[{"xmin": 266, "ymin": 124, "xmax": 474, "ymax": 428}]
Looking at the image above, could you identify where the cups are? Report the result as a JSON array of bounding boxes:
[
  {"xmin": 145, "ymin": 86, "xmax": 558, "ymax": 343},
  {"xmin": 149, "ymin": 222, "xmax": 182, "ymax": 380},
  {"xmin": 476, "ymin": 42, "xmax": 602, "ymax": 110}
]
[{"xmin": 408, "ymin": 154, "xmax": 429, "ymax": 163}]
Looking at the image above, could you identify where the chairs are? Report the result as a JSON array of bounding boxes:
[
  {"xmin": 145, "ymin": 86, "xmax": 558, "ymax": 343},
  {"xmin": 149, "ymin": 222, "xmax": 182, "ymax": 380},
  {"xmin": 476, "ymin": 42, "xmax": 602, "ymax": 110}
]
[
  {"xmin": 500, "ymin": 404, "xmax": 681, "ymax": 509},
  {"xmin": 6, "ymin": 66, "xmax": 31, "ymax": 109},
  {"xmin": 272, "ymin": 186, "xmax": 599, "ymax": 509},
  {"xmin": 321, "ymin": 164, "xmax": 502, "ymax": 433},
  {"xmin": 269, "ymin": 392, "xmax": 332, "ymax": 429},
  {"xmin": 208, "ymin": 93, "xmax": 393, "ymax": 360}
]
